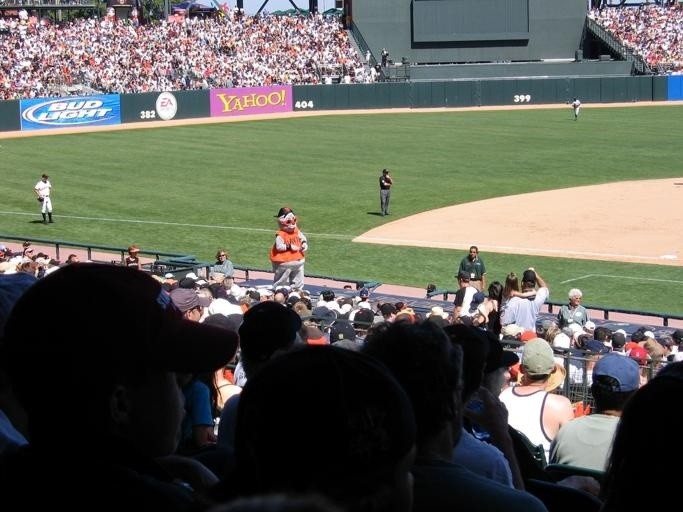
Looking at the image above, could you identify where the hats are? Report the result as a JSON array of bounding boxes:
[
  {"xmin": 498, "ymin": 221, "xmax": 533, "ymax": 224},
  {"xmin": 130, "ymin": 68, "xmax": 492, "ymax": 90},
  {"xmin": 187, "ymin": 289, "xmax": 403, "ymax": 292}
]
[
  {"xmin": 522, "ymin": 270, "xmax": 537, "ymax": 283},
  {"xmin": 467, "ymin": 334, "xmax": 519, "ymax": 373},
  {"xmin": 234, "ymin": 343, "xmax": 419, "ymax": 487},
  {"xmin": 383, "ymin": 169, "xmax": 390, "ymax": 173},
  {"xmin": 130, "ymin": 247, "xmax": 310, "ymax": 314},
  {"xmin": 353, "ymin": 308, "xmax": 374, "ymax": 330},
  {"xmin": 670, "ymin": 330, "xmax": 683, "ymax": 342},
  {"xmin": 381, "ymin": 302, "xmax": 397, "ymax": 316},
  {"xmin": 471, "ymin": 292, "xmax": 484, "ymax": 311},
  {"xmin": 0, "ymin": 261, "xmax": 238, "ymax": 407},
  {"xmin": 516, "ymin": 337, "xmax": 556, "ymax": 376},
  {"xmin": 592, "ymin": 355, "xmax": 624, "ymax": 393},
  {"xmin": 612, "ymin": 334, "xmax": 625, "ymax": 348},
  {"xmin": 311, "ymin": 307, "xmax": 338, "ymax": 331}
]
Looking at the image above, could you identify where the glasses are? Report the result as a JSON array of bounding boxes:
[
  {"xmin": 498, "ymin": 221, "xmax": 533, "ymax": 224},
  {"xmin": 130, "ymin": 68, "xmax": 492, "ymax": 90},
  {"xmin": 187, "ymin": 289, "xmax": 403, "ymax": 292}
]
[{"xmin": 218, "ymin": 254, "xmax": 225, "ymax": 258}]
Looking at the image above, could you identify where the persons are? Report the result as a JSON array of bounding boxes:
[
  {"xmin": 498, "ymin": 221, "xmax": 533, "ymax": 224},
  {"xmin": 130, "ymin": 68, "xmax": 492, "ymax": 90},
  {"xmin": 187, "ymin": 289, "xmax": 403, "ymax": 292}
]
[
  {"xmin": 572, "ymin": 97, "xmax": 580, "ymax": 120},
  {"xmin": 0, "ymin": 4, "xmax": 388, "ymax": 98},
  {"xmin": 0, "ymin": 249, "xmax": 682, "ymax": 512},
  {"xmin": 380, "ymin": 168, "xmax": 393, "ymax": 216},
  {"xmin": 35, "ymin": 173, "xmax": 54, "ymax": 224},
  {"xmin": 589, "ymin": 1, "xmax": 683, "ymax": 70}
]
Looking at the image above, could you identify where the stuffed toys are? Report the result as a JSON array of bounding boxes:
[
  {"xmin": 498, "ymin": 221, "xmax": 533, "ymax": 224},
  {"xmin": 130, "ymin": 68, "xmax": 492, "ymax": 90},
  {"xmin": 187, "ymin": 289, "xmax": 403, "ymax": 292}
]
[{"xmin": 270, "ymin": 208, "xmax": 308, "ymax": 290}]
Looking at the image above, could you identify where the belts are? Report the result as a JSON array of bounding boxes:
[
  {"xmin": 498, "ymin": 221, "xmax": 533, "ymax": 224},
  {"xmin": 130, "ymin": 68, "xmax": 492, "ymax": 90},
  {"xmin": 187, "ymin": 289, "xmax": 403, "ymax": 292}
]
[{"xmin": 470, "ymin": 278, "xmax": 481, "ymax": 282}]
